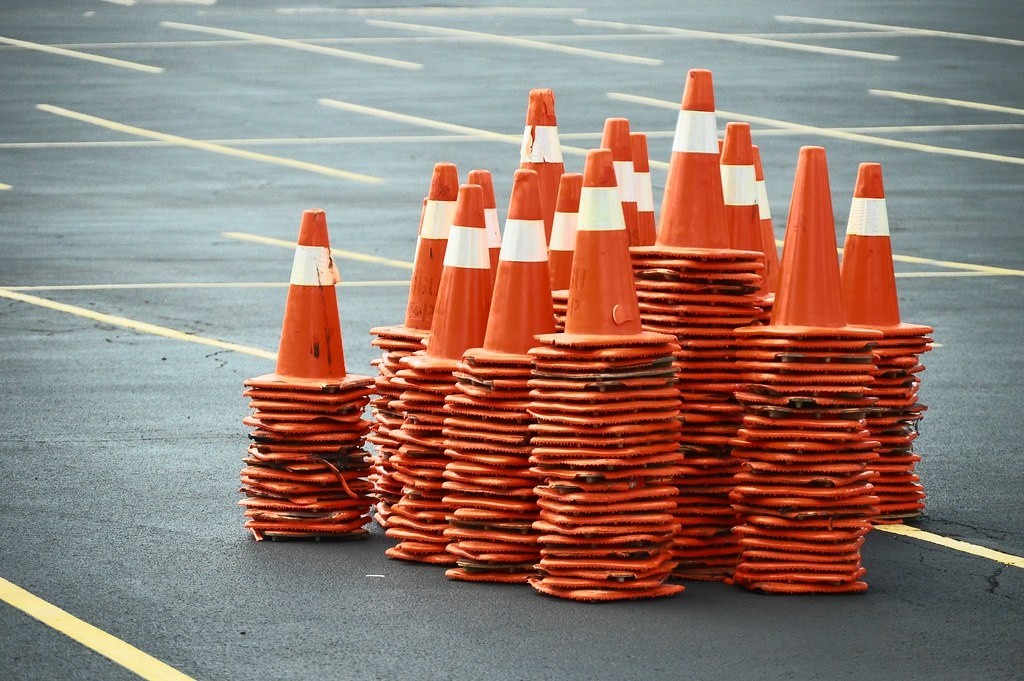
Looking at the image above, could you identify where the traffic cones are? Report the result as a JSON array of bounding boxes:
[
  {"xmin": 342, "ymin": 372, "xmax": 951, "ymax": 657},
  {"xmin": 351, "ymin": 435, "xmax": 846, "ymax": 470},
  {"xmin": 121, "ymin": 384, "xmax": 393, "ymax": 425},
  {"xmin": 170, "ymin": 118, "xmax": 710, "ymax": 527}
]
[{"xmin": 239, "ymin": 68, "xmax": 934, "ymax": 603}]
